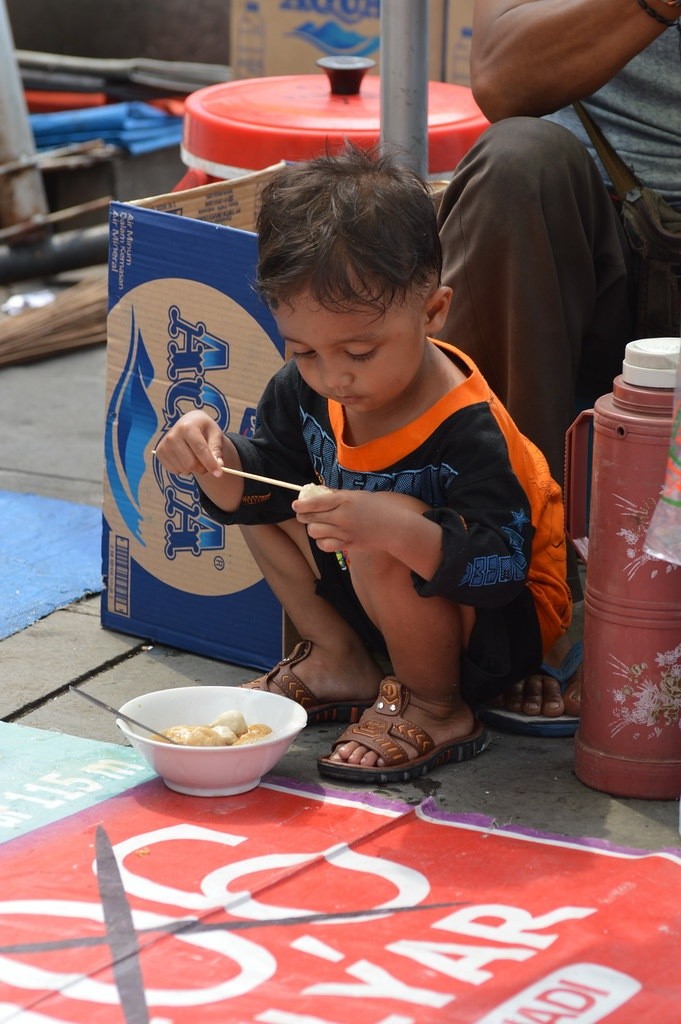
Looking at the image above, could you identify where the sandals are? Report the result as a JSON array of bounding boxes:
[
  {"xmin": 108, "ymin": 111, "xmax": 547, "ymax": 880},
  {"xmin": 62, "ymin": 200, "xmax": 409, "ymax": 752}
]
[{"xmin": 482, "ymin": 642, "xmax": 582, "ymax": 738}]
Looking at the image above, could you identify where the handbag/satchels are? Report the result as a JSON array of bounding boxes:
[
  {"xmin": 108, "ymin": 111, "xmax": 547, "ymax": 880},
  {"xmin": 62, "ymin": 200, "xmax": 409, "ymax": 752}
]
[{"xmin": 621, "ymin": 186, "xmax": 681, "ymax": 339}]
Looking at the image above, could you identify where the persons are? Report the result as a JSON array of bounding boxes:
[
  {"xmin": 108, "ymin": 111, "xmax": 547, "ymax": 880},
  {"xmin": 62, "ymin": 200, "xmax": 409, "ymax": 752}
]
[
  {"xmin": 430, "ymin": 0, "xmax": 681, "ymax": 735},
  {"xmin": 156, "ymin": 139, "xmax": 573, "ymax": 784}
]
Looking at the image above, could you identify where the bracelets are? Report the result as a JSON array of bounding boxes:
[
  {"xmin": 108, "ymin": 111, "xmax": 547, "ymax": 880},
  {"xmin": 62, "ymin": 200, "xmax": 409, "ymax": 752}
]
[{"xmin": 637, "ymin": 0, "xmax": 679, "ymax": 26}]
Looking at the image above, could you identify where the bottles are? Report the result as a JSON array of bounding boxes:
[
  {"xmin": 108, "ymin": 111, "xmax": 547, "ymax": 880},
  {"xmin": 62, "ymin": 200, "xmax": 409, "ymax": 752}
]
[{"xmin": 563, "ymin": 335, "xmax": 681, "ymax": 802}]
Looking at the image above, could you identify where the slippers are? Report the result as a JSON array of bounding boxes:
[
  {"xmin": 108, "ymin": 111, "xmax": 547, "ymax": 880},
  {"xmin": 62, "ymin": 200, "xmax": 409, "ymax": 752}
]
[
  {"xmin": 315, "ymin": 678, "xmax": 486, "ymax": 779},
  {"xmin": 236, "ymin": 639, "xmax": 378, "ymax": 726}
]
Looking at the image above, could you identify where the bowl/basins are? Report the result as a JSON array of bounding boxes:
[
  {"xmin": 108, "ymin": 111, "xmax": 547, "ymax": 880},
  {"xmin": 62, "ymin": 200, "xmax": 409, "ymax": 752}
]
[{"xmin": 116, "ymin": 686, "xmax": 308, "ymax": 797}]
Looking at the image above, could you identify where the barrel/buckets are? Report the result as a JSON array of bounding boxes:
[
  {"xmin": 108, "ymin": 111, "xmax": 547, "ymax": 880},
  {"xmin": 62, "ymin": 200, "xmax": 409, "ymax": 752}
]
[{"xmin": 169, "ymin": 53, "xmax": 493, "ymax": 191}]
